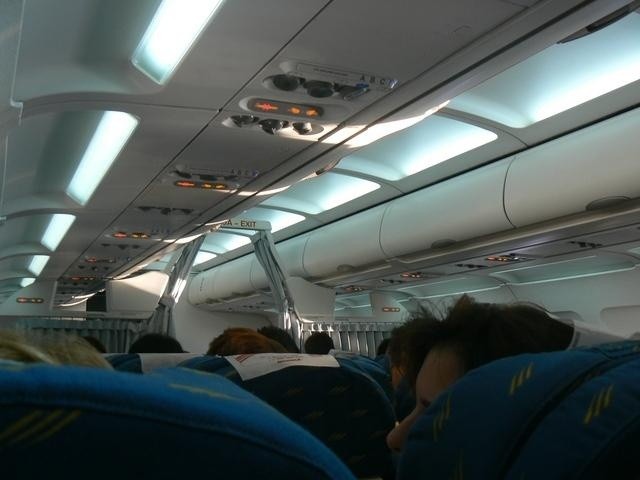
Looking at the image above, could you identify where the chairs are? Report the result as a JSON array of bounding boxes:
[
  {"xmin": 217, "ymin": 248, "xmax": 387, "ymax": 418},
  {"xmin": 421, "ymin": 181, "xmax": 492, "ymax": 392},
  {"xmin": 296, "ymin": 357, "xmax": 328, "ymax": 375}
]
[{"xmin": 1, "ymin": 341, "xmax": 640, "ymax": 480}]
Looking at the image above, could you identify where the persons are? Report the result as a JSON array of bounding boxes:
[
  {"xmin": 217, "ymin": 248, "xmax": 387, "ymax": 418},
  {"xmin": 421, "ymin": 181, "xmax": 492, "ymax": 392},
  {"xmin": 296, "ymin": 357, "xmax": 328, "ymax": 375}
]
[
  {"xmin": 383, "ymin": 315, "xmax": 442, "ymax": 393},
  {"xmin": 375, "ymin": 336, "xmax": 393, "ymax": 357},
  {"xmin": 205, "ymin": 327, "xmax": 287, "ymax": 356},
  {"xmin": 128, "ymin": 330, "xmax": 186, "ymax": 354},
  {"xmin": 255, "ymin": 324, "xmax": 302, "ymax": 354},
  {"xmin": 303, "ymin": 330, "xmax": 337, "ymax": 355},
  {"xmin": 385, "ymin": 300, "xmax": 577, "ymax": 455}
]
